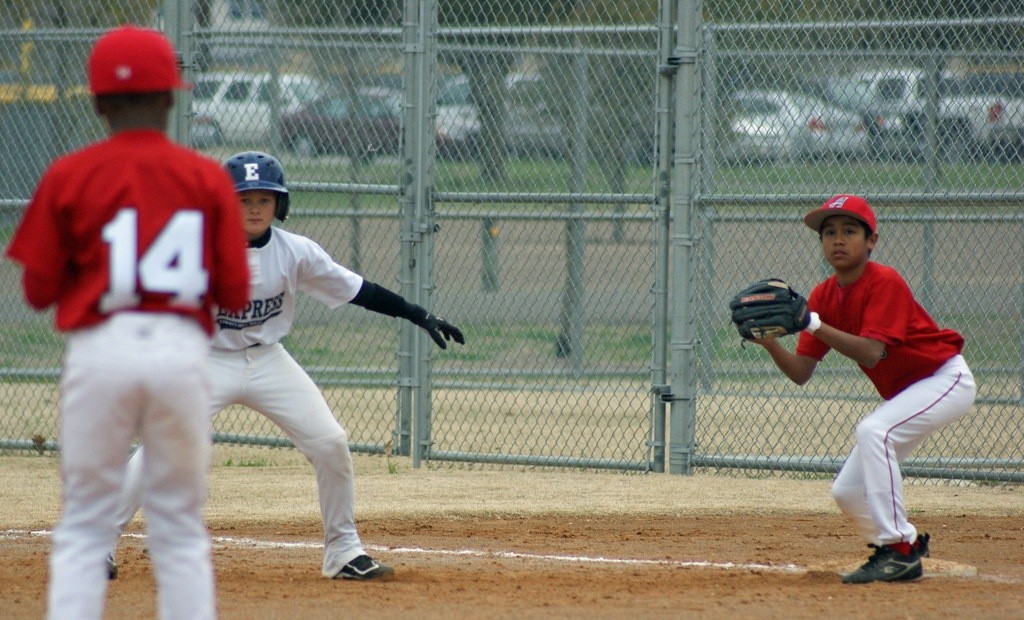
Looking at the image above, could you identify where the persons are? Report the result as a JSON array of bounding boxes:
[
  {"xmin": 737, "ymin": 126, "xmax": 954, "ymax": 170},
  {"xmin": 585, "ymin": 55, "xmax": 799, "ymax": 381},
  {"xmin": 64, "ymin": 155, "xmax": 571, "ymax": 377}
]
[
  {"xmin": 103, "ymin": 150, "xmax": 467, "ymax": 580},
  {"xmin": 729, "ymin": 195, "xmax": 975, "ymax": 584},
  {"xmin": 5, "ymin": 29, "xmax": 250, "ymax": 620}
]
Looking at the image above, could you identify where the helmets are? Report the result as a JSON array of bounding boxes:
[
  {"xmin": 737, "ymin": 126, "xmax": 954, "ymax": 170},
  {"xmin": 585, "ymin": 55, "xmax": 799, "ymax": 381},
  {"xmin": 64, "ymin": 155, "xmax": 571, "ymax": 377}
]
[{"xmin": 221, "ymin": 150, "xmax": 289, "ymax": 222}]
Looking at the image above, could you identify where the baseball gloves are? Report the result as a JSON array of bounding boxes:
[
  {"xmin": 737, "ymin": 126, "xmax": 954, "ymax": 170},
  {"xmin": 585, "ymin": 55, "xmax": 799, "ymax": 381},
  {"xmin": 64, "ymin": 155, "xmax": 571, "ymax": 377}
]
[{"xmin": 728, "ymin": 277, "xmax": 811, "ymax": 341}]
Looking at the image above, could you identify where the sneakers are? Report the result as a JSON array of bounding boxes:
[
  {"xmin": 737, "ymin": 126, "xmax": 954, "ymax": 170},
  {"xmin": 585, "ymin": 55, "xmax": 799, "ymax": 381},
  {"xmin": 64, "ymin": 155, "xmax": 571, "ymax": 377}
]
[
  {"xmin": 843, "ymin": 544, "xmax": 921, "ymax": 582},
  {"xmin": 331, "ymin": 555, "xmax": 397, "ymax": 581},
  {"xmin": 107, "ymin": 558, "xmax": 118, "ymax": 580},
  {"xmin": 918, "ymin": 533, "xmax": 931, "ymax": 558}
]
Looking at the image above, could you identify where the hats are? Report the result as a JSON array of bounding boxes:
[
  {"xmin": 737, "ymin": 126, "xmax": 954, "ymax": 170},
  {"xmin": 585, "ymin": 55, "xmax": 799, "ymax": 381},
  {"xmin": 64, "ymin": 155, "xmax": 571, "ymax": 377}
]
[
  {"xmin": 805, "ymin": 196, "xmax": 876, "ymax": 233},
  {"xmin": 90, "ymin": 27, "xmax": 189, "ymax": 91}
]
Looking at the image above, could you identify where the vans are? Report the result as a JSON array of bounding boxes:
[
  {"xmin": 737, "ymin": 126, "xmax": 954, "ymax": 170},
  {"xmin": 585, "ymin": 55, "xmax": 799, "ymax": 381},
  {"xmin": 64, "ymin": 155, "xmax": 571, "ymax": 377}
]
[{"xmin": 195, "ymin": 72, "xmax": 342, "ymax": 145}]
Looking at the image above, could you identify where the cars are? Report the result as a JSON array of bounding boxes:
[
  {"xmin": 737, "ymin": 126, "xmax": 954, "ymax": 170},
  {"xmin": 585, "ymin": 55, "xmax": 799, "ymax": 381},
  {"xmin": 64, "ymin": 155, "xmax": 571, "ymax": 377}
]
[
  {"xmin": 436, "ymin": 69, "xmax": 564, "ymax": 146},
  {"xmin": 830, "ymin": 65, "xmax": 1024, "ymax": 161},
  {"xmin": 276, "ymin": 97, "xmax": 469, "ymax": 163},
  {"xmin": 722, "ymin": 87, "xmax": 872, "ymax": 160}
]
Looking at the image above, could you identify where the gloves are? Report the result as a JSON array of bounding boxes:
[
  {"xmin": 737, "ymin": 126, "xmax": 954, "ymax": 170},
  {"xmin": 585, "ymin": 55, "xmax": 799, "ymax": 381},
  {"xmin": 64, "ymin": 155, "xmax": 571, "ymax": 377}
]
[{"xmin": 408, "ymin": 304, "xmax": 465, "ymax": 349}]
